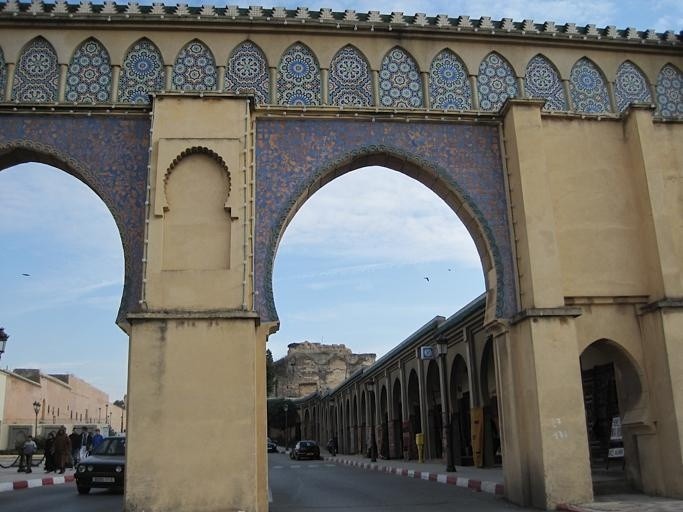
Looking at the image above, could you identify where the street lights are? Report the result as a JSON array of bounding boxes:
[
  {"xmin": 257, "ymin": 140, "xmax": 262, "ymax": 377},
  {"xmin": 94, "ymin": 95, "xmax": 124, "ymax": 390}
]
[
  {"xmin": 364, "ymin": 379, "xmax": 377, "ymax": 461},
  {"xmin": 435, "ymin": 332, "xmax": 456, "ymax": 472},
  {"xmin": 327, "ymin": 397, "xmax": 337, "ymax": 456},
  {"xmin": 282, "ymin": 402, "xmax": 288, "ymax": 450},
  {"xmin": 0, "ymin": 326, "xmax": 9, "ymax": 360},
  {"xmin": 31, "ymin": 401, "xmax": 41, "ymax": 454}
]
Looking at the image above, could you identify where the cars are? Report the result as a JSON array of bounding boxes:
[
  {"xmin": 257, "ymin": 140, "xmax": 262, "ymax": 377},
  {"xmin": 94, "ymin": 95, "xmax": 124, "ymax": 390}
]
[
  {"xmin": 267, "ymin": 437, "xmax": 277, "ymax": 452},
  {"xmin": 289, "ymin": 439, "xmax": 320, "ymax": 460},
  {"xmin": 72, "ymin": 436, "xmax": 125, "ymax": 495}
]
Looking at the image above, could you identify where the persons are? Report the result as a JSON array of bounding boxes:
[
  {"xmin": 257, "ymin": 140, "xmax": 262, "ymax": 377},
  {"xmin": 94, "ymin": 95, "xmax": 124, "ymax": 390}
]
[
  {"xmin": 20, "ymin": 435, "xmax": 38, "ymax": 474},
  {"xmin": 42, "ymin": 426, "xmax": 102, "ymax": 473}
]
